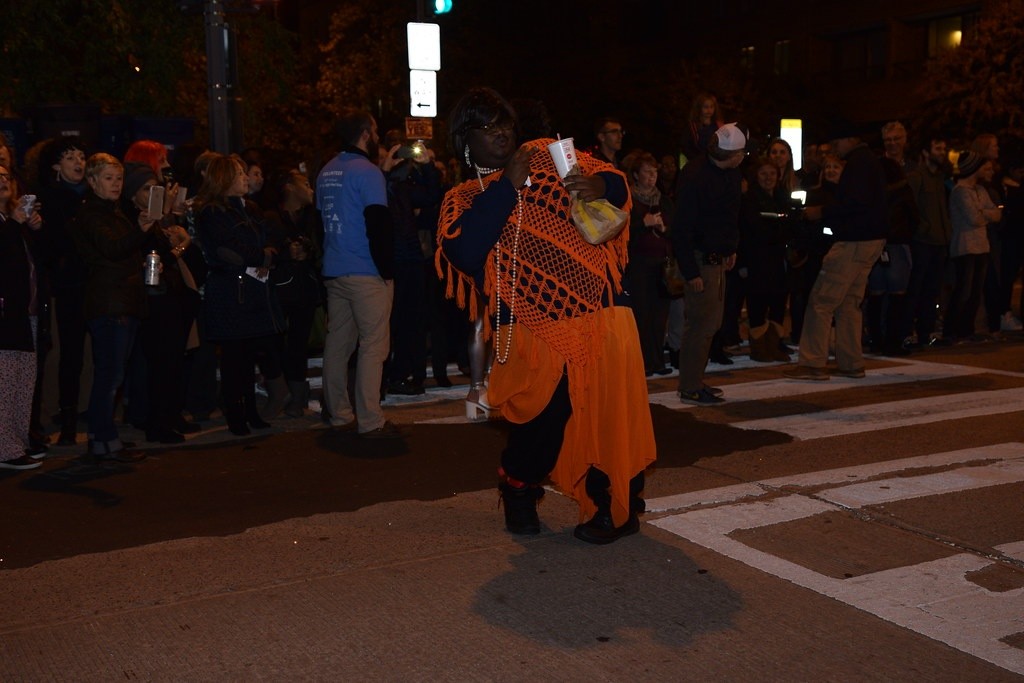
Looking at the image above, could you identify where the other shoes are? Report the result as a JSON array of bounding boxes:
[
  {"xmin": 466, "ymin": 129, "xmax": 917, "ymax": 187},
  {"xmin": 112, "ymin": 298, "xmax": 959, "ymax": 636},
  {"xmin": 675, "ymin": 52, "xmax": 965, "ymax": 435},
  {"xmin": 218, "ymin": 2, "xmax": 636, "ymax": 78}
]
[
  {"xmin": 384, "ymin": 360, "xmax": 471, "ymax": 394},
  {"xmin": 642, "ymin": 329, "xmax": 796, "ymax": 377},
  {"xmin": 782, "ymin": 365, "xmax": 829, "ymax": 380},
  {"xmin": 335, "ymin": 417, "xmax": 359, "ymax": 431},
  {"xmin": 830, "ymin": 365, "xmax": 866, "ymax": 378},
  {"xmin": 361, "ymin": 421, "xmax": 402, "ymax": 439},
  {"xmin": 0, "ymin": 383, "xmax": 310, "ymax": 469},
  {"xmin": 147, "ymin": 426, "xmax": 186, "ymax": 444},
  {"xmin": 170, "ymin": 416, "xmax": 202, "ymax": 433},
  {"xmin": 864, "ymin": 315, "xmax": 1024, "ymax": 356}
]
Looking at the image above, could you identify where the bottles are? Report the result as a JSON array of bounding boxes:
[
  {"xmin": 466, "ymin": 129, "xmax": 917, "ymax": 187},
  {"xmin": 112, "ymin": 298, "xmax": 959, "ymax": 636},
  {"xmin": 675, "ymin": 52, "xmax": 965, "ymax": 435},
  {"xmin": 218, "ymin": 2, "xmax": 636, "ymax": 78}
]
[{"xmin": 145, "ymin": 250, "xmax": 160, "ymax": 285}]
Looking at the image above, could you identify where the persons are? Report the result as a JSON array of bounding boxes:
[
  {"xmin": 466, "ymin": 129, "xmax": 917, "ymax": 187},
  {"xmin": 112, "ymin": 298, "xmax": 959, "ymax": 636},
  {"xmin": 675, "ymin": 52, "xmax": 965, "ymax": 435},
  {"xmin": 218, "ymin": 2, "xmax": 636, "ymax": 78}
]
[
  {"xmin": 435, "ymin": 87, "xmax": 657, "ymax": 546},
  {"xmin": 0, "ymin": 89, "xmax": 1024, "ymax": 471},
  {"xmin": 670, "ymin": 124, "xmax": 747, "ymax": 407}
]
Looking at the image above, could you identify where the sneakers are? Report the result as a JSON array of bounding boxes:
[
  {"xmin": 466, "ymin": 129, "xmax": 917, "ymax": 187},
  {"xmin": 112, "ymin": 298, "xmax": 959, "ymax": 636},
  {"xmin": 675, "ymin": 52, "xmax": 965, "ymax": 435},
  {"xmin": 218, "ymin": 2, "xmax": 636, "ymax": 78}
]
[
  {"xmin": 702, "ymin": 384, "xmax": 723, "ymax": 397},
  {"xmin": 576, "ymin": 507, "xmax": 641, "ymax": 545},
  {"xmin": 681, "ymin": 388, "xmax": 727, "ymax": 406},
  {"xmin": 503, "ymin": 490, "xmax": 541, "ymax": 534}
]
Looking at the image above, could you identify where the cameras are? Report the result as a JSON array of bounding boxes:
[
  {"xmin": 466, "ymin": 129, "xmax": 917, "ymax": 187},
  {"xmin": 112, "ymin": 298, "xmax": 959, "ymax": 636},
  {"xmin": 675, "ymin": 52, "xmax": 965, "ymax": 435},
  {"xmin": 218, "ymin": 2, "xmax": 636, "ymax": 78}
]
[{"xmin": 296, "ymin": 237, "xmax": 318, "ymax": 259}]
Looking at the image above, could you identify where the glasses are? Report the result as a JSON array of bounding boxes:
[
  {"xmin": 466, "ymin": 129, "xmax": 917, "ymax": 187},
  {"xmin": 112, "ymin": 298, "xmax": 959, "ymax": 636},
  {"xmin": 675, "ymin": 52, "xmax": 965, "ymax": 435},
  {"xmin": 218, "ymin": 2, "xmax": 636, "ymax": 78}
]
[
  {"xmin": 474, "ymin": 118, "xmax": 514, "ymax": 135},
  {"xmin": 602, "ymin": 128, "xmax": 626, "ymax": 134},
  {"xmin": 0, "ymin": 173, "xmax": 13, "ymax": 181}
]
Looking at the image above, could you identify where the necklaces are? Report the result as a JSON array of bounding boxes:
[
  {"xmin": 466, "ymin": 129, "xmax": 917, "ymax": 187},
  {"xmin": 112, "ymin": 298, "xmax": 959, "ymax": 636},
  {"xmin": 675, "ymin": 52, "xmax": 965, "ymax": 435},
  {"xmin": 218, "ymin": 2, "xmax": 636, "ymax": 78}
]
[{"xmin": 464, "ymin": 143, "xmax": 523, "ymax": 364}]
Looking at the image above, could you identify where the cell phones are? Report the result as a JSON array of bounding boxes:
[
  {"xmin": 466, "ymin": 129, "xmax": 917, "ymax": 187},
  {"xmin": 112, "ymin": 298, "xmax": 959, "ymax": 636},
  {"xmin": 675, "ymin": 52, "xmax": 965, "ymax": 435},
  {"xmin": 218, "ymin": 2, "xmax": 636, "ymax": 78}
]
[
  {"xmin": 650, "ymin": 205, "xmax": 660, "ymax": 214},
  {"xmin": 20, "ymin": 195, "xmax": 36, "ymax": 218},
  {"xmin": 397, "ymin": 145, "xmax": 419, "ymax": 158},
  {"xmin": 148, "ymin": 186, "xmax": 164, "ymax": 220}
]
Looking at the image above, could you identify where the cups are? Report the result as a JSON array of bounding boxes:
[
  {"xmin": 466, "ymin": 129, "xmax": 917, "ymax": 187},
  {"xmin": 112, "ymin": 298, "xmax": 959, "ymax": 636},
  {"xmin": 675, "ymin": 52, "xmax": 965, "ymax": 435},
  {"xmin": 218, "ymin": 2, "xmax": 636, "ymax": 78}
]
[{"xmin": 547, "ymin": 137, "xmax": 580, "ymax": 187}]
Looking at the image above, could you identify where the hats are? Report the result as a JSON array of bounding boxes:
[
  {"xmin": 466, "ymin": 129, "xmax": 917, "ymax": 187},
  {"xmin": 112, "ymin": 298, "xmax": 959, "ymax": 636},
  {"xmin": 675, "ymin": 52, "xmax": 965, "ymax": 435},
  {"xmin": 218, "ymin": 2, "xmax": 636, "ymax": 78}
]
[
  {"xmin": 122, "ymin": 162, "xmax": 159, "ymax": 198},
  {"xmin": 958, "ymin": 151, "xmax": 986, "ymax": 176},
  {"xmin": 828, "ymin": 115, "xmax": 863, "ymax": 141},
  {"xmin": 714, "ymin": 121, "xmax": 746, "ymax": 150}
]
